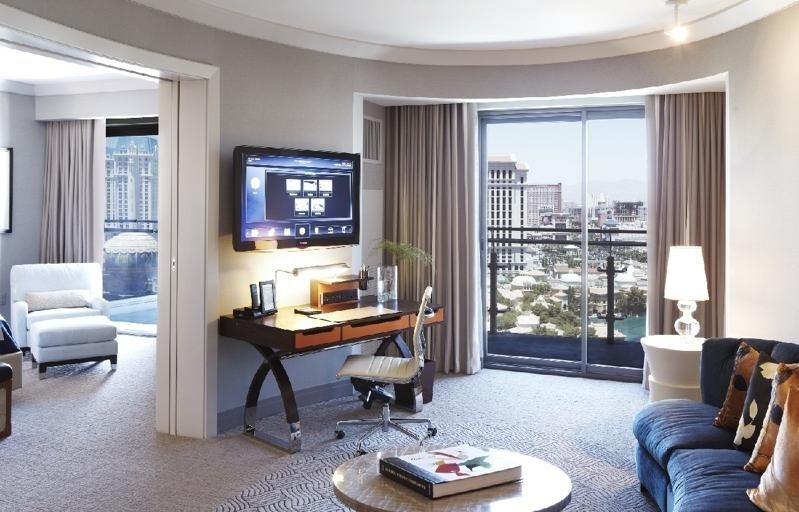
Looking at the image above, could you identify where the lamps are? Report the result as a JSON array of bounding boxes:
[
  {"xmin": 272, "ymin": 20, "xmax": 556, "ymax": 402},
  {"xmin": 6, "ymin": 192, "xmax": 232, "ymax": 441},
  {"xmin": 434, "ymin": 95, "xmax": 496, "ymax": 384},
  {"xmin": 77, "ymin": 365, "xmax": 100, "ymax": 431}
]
[
  {"xmin": 664, "ymin": 244, "xmax": 711, "ymax": 338},
  {"xmin": 275, "ymin": 263, "xmax": 350, "ymax": 299}
]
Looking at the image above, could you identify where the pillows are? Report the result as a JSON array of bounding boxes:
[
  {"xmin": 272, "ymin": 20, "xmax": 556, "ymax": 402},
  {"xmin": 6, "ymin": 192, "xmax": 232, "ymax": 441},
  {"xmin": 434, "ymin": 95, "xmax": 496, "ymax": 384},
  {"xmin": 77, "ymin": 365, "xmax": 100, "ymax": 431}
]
[
  {"xmin": 734, "ymin": 352, "xmax": 799, "ymax": 452},
  {"xmin": 745, "ymin": 384, "xmax": 799, "ymax": 509},
  {"xmin": 715, "ymin": 342, "xmax": 756, "ymax": 425},
  {"xmin": 27, "ymin": 287, "xmax": 94, "ymax": 308},
  {"xmin": 745, "ymin": 363, "xmax": 799, "ymax": 473}
]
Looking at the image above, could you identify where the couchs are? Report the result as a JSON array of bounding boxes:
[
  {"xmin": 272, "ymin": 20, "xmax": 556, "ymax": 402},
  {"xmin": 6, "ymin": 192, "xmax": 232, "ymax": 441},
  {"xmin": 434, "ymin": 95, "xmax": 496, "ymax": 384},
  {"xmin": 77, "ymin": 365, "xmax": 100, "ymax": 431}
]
[{"xmin": 631, "ymin": 337, "xmax": 799, "ymax": 510}]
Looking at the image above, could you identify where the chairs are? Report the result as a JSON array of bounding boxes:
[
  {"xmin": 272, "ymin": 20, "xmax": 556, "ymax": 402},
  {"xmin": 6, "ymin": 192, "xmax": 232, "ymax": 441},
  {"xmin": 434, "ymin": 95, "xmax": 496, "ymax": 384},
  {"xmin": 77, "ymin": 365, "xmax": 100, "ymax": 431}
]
[
  {"xmin": 12, "ymin": 259, "xmax": 110, "ymax": 354},
  {"xmin": 332, "ymin": 288, "xmax": 439, "ymax": 455}
]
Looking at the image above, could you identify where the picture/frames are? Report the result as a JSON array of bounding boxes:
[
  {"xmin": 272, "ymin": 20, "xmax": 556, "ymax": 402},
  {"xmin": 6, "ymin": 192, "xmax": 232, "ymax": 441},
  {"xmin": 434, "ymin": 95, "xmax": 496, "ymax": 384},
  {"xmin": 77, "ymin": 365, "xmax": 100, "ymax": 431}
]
[
  {"xmin": 2, "ymin": 148, "xmax": 14, "ymax": 234},
  {"xmin": 256, "ymin": 280, "xmax": 277, "ymax": 315}
]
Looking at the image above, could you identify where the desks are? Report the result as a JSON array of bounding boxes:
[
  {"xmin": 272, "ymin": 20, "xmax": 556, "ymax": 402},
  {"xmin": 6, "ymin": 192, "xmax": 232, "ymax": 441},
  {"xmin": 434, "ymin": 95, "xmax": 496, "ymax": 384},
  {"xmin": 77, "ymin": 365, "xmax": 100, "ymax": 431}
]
[{"xmin": 219, "ymin": 300, "xmax": 445, "ymax": 454}]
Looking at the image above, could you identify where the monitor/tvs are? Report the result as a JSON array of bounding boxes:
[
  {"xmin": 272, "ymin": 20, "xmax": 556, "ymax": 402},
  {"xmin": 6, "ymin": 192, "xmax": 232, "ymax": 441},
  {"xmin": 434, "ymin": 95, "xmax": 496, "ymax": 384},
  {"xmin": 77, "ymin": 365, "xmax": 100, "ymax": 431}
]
[{"xmin": 233, "ymin": 146, "xmax": 360, "ymax": 251}]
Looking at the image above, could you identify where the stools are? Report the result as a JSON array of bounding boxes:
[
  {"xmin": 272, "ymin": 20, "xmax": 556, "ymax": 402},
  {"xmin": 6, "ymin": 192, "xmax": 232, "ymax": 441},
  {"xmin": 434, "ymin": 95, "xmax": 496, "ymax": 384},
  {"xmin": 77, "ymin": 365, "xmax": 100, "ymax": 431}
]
[
  {"xmin": 0, "ymin": 363, "xmax": 12, "ymax": 438},
  {"xmin": 27, "ymin": 317, "xmax": 119, "ymax": 375}
]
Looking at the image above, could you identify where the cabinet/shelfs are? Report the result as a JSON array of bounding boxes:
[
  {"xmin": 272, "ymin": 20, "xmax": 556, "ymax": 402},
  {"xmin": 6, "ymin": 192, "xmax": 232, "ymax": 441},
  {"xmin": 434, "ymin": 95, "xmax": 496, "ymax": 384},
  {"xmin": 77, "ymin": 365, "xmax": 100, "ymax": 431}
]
[{"xmin": 639, "ymin": 336, "xmax": 701, "ymax": 403}]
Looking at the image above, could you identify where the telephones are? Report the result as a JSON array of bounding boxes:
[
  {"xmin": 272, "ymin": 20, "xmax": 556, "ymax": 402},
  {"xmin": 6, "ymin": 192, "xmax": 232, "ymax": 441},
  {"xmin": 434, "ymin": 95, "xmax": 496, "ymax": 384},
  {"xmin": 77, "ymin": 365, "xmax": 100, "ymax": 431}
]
[{"xmin": 244, "ymin": 284, "xmax": 262, "ymax": 319}]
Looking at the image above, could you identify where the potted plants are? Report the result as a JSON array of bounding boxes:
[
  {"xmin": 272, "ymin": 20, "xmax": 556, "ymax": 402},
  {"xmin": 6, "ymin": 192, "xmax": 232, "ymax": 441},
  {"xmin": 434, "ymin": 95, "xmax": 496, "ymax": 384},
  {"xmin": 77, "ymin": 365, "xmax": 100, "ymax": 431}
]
[{"xmin": 374, "ymin": 239, "xmax": 434, "ymax": 302}]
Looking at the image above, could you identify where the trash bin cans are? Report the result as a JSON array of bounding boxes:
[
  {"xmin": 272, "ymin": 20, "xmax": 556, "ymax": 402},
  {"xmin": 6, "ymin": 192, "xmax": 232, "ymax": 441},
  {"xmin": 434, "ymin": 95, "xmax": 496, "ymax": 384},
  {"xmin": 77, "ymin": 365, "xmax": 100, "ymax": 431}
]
[{"xmin": 395, "ymin": 359, "xmax": 436, "ymax": 407}]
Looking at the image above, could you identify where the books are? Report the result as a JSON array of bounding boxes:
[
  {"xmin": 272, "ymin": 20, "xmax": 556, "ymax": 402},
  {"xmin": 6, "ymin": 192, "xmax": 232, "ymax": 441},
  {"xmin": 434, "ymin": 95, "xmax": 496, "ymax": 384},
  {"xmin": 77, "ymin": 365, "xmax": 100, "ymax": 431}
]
[
  {"xmin": 379, "ymin": 446, "xmax": 524, "ymax": 500},
  {"xmin": 294, "ymin": 306, "xmax": 321, "ymax": 316},
  {"xmin": 308, "ymin": 305, "xmax": 403, "ymax": 324}
]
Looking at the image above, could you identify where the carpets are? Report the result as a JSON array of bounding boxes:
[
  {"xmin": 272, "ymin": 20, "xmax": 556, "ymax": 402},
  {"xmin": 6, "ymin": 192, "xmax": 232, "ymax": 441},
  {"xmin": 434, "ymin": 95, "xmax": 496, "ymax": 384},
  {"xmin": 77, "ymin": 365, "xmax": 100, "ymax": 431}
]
[{"xmin": 214, "ymin": 420, "xmax": 662, "ymax": 512}]
